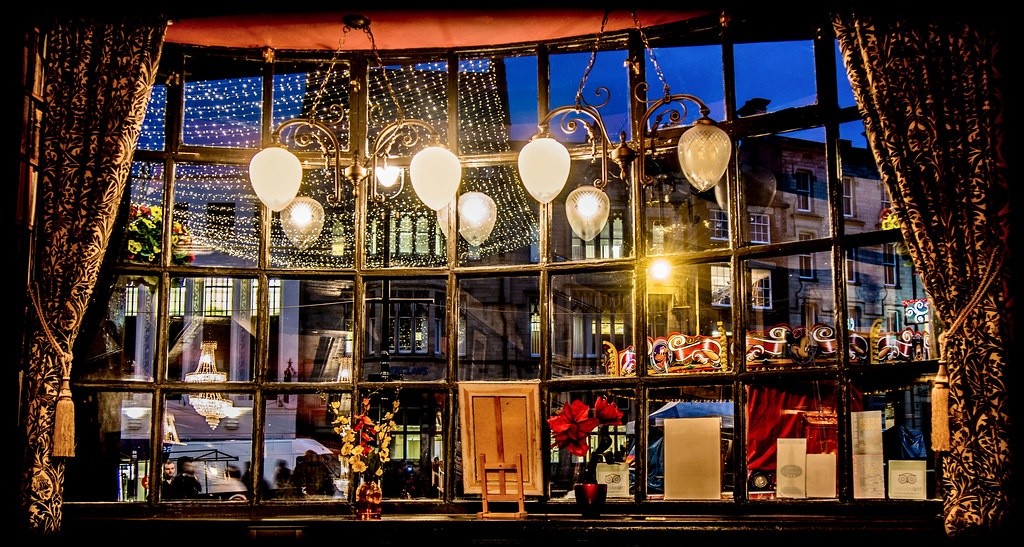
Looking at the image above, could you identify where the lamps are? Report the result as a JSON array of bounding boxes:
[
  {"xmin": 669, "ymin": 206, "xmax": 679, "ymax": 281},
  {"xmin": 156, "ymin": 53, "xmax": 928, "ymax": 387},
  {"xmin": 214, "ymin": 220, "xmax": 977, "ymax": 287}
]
[
  {"xmin": 248, "ymin": 13, "xmax": 464, "ymax": 214},
  {"xmin": 278, "ymin": 108, "xmax": 729, "ymax": 253},
  {"xmin": 517, "ymin": 0, "xmax": 732, "ymax": 205},
  {"xmin": 120, "ymin": 340, "xmax": 252, "ymax": 446}
]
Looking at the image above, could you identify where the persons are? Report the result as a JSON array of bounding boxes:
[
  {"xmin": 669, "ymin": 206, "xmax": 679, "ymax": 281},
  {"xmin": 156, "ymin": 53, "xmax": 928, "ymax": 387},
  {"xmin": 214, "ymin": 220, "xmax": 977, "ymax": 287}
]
[
  {"xmin": 161, "ymin": 456, "xmax": 202, "ymax": 500},
  {"xmin": 241, "ymin": 460, "xmax": 252, "ymax": 494},
  {"xmin": 274, "ymin": 450, "xmax": 334, "ymax": 495}
]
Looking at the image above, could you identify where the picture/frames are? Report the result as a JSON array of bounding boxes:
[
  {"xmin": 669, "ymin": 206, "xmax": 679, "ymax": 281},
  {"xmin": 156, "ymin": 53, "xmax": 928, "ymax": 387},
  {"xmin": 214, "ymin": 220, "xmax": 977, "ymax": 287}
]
[{"xmin": 458, "ymin": 381, "xmax": 544, "ymax": 495}]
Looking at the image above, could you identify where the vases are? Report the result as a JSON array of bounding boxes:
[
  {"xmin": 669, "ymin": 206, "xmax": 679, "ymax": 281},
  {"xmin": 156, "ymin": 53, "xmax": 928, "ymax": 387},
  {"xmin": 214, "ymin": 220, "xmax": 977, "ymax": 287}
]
[
  {"xmin": 574, "ymin": 484, "xmax": 607, "ymax": 520},
  {"xmin": 356, "ymin": 481, "xmax": 382, "ymax": 520}
]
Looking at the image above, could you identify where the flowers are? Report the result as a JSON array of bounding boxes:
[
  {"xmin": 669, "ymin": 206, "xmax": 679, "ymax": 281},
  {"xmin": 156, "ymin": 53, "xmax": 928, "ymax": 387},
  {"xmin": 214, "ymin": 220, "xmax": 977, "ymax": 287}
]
[
  {"xmin": 316, "ymin": 384, "xmax": 402, "ymax": 485},
  {"xmin": 546, "ymin": 395, "xmax": 625, "ymax": 484},
  {"xmin": 116, "ymin": 203, "xmax": 198, "ymax": 303}
]
